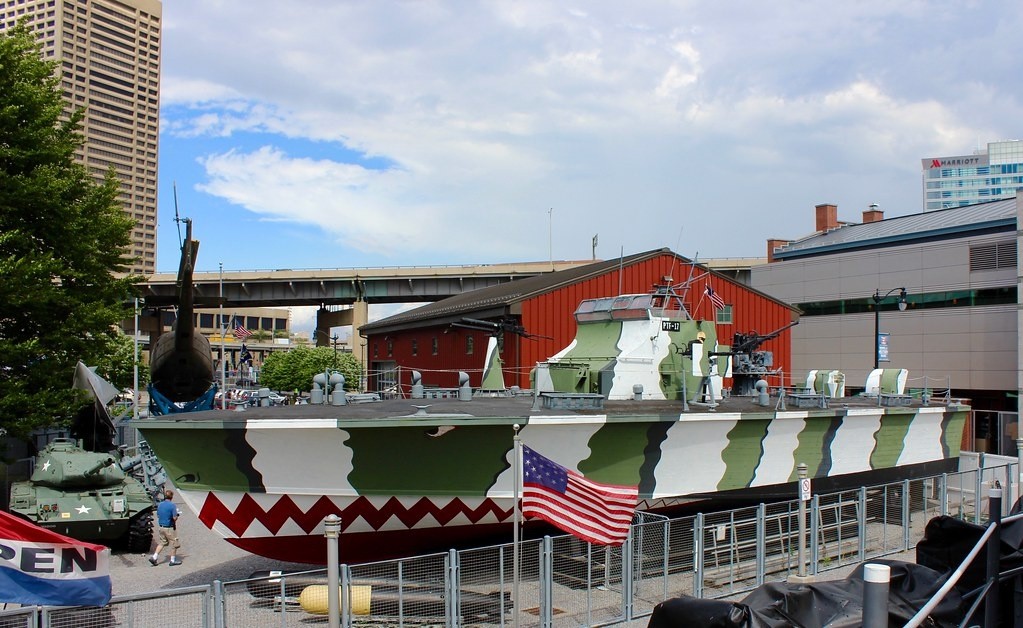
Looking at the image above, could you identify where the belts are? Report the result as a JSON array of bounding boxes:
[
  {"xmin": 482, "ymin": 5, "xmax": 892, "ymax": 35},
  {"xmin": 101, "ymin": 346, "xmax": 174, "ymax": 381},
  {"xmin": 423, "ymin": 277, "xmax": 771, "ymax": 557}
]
[{"xmin": 160, "ymin": 525, "xmax": 170, "ymax": 528}]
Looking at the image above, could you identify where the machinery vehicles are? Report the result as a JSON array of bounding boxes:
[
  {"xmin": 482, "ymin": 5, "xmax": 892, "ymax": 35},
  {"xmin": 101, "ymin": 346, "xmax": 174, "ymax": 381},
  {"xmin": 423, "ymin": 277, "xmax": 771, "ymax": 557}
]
[{"xmin": 8, "ymin": 438, "xmax": 157, "ymax": 555}]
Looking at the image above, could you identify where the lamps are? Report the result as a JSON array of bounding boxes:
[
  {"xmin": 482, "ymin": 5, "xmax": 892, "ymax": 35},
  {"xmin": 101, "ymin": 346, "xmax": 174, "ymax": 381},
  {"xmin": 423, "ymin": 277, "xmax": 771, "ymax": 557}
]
[{"xmin": 662, "ymin": 276, "xmax": 674, "ymax": 282}]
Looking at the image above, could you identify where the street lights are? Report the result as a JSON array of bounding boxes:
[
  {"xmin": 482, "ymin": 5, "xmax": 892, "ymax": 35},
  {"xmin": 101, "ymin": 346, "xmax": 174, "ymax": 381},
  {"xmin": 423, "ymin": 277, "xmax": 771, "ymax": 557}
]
[
  {"xmin": 312, "ymin": 330, "xmax": 339, "ymax": 368},
  {"xmin": 871, "ymin": 286, "xmax": 908, "ymax": 371}
]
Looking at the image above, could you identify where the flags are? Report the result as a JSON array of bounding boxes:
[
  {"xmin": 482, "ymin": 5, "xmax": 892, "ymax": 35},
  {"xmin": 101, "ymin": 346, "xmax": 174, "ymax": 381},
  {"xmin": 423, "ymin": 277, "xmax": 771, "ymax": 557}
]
[
  {"xmin": 240, "ymin": 344, "xmax": 252, "ymax": 363},
  {"xmin": 0, "ymin": 510, "xmax": 112, "ymax": 606},
  {"xmin": 522, "ymin": 442, "xmax": 639, "ymax": 547},
  {"xmin": 235, "ymin": 319, "xmax": 252, "ymax": 340},
  {"xmin": 704, "ymin": 284, "xmax": 725, "ymax": 311}
]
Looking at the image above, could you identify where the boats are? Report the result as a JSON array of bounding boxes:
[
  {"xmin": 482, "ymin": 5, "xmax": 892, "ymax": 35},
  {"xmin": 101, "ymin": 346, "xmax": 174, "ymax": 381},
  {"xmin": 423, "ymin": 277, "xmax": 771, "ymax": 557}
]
[{"xmin": 124, "ymin": 217, "xmax": 976, "ymax": 574}]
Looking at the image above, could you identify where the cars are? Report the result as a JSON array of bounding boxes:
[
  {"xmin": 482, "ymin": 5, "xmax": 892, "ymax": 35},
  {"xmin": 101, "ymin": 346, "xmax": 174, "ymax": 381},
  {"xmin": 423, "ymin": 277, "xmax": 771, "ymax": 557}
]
[{"xmin": 118, "ymin": 388, "xmax": 141, "ymax": 402}]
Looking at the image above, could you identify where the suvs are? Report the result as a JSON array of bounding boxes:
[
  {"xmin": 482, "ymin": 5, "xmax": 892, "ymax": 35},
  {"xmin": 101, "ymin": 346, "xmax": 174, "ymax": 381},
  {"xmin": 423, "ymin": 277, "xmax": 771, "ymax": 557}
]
[{"xmin": 214, "ymin": 389, "xmax": 287, "ymax": 407}]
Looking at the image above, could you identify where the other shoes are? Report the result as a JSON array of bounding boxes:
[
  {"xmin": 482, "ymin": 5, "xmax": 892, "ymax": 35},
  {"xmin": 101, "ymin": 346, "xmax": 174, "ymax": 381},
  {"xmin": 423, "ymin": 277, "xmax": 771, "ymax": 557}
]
[
  {"xmin": 169, "ymin": 560, "xmax": 182, "ymax": 566},
  {"xmin": 149, "ymin": 557, "xmax": 158, "ymax": 566}
]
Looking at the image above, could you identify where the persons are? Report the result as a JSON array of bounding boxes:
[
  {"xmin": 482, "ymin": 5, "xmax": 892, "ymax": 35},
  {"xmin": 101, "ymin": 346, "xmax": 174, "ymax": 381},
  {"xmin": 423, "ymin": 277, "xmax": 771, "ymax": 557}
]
[
  {"xmin": 149, "ymin": 490, "xmax": 182, "ymax": 566},
  {"xmin": 209, "ymin": 383, "xmax": 254, "ymax": 407},
  {"xmin": 285, "ymin": 393, "xmax": 302, "ymax": 405}
]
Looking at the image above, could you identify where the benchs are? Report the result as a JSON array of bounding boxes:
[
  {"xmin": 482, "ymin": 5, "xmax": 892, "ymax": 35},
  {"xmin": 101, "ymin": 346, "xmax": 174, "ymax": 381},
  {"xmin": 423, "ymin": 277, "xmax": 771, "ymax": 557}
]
[{"xmin": 769, "ymin": 386, "xmax": 811, "ymax": 398}]
